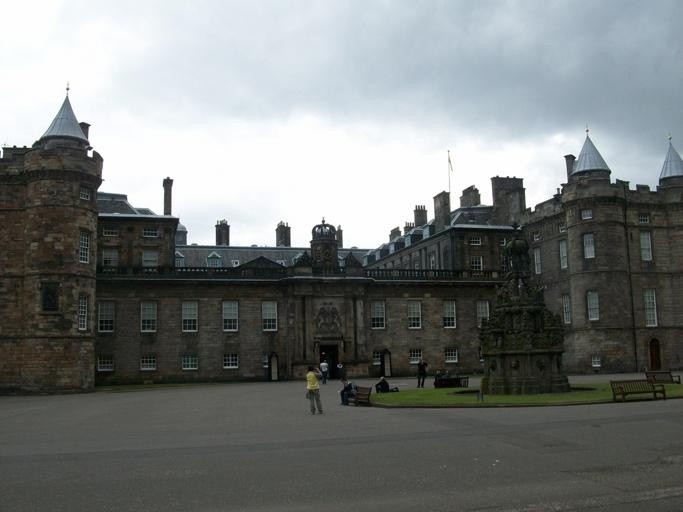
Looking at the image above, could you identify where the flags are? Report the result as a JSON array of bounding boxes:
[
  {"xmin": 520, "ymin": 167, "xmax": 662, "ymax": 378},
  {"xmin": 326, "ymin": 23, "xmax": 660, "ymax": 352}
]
[{"xmin": 448, "ymin": 156, "xmax": 454, "ymax": 171}]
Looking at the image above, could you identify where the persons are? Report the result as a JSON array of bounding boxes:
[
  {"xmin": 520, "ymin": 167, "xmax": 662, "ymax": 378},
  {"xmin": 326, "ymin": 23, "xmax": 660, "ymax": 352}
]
[
  {"xmin": 416, "ymin": 359, "xmax": 428, "ymax": 389},
  {"xmin": 306, "ymin": 365, "xmax": 324, "ymax": 416},
  {"xmin": 339, "ymin": 378, "xmax": 355, "ymax": 406},
  {"xmin": 319, "ymin": 359, "xmax": 329, "ymax": 385},
  {"xmin": 375, "ymin": 376, "xmax": 389, "ymax": 394}
]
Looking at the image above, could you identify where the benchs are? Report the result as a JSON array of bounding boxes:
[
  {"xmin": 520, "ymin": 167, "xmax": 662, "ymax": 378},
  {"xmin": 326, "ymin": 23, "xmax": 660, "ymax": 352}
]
[
  {"xmin": 341, "ymin": 387, "xmax": 371, "ymax": 407},
  {"xmin": 646, "ymin": 372, "xmax": 680, "ymax": 384},
  {"xmin": 611, "ymin": 380, "xmax": 664, "ymax": 402}
]
